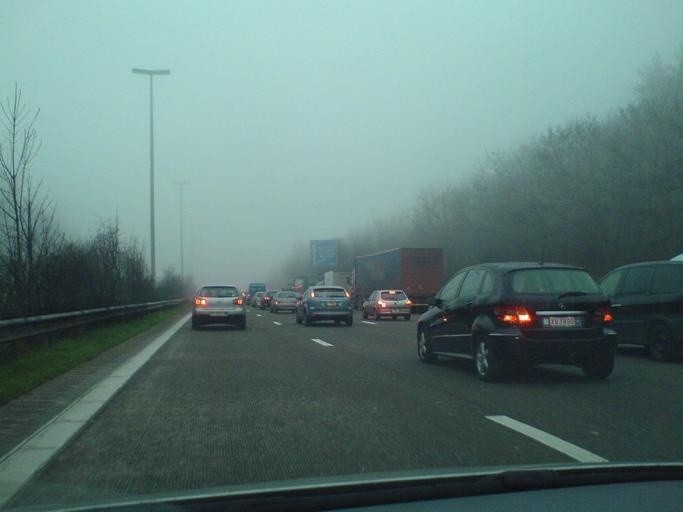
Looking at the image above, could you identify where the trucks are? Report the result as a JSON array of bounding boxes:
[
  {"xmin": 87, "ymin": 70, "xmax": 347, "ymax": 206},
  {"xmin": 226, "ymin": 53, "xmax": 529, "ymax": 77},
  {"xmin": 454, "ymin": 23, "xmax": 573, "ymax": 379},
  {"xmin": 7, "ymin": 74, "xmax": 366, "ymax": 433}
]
[{"xmin": 348, "ymin": 250, "xmax": 443, "ymax": 309}]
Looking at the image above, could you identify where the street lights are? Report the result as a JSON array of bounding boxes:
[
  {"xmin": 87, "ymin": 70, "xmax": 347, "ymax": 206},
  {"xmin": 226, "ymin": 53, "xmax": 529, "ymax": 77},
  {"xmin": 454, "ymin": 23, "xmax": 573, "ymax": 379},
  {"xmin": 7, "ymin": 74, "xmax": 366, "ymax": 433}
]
[{"xmin": 131, "ymin": 68, "xmax": 171, "ymax": 285}]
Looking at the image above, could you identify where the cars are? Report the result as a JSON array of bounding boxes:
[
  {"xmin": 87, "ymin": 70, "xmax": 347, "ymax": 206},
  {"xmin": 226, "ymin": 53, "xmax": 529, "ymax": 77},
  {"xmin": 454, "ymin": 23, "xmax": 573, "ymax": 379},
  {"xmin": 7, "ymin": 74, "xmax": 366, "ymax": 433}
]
[
  {"xmin": 244, "ymin": 271, "xmax": 352, "ymax": 325},
  {"xmin": 416, "ymin": 252, "xmax": 683, "ymax": 383},
  {"xmin": 192, "ymin": 284, "xmax": 244, "ymax": 330},
  {"xmin": 359, "ymin": 289, "xmax": 413, "ymax": 321}
]
